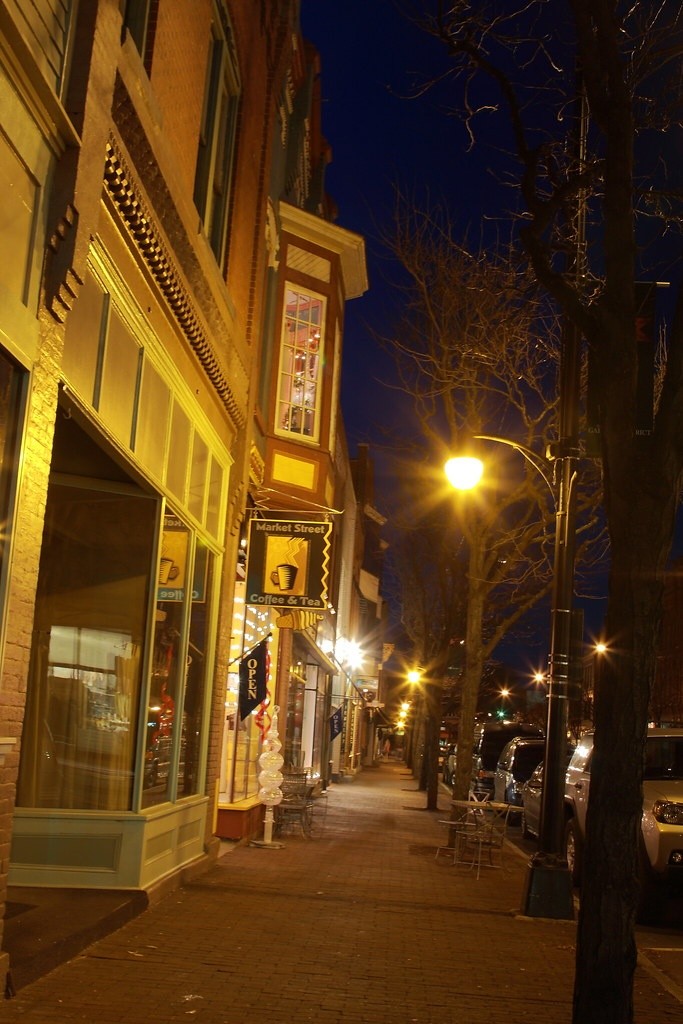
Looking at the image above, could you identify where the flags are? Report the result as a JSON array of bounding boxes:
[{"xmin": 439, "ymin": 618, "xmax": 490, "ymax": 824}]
[
  {"xmin": 239, "ymin": 640, "xmax": 266, "ymax": 722},
  {"xmin": 329, "ymin": 708, "xmax": 343, "ymax": 741}
]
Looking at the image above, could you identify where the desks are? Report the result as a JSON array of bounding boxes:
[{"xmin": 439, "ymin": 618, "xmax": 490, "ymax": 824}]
[{"xmin": 449, "ymin": 800, "xmax": 524, "ymax": 869}]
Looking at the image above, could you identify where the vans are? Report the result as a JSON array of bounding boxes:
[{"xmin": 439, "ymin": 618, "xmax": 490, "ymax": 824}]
[
  {"xmin": 472, "ymin": 719, "xmax": 544, "ymax": 802},
  {"xmin": 492, "ymin": 735, "xmax": 578, "ymax": 821}
]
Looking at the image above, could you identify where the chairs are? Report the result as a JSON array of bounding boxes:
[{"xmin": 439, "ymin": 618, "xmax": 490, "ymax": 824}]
[
  {"xmin": 272, "ymin": 772, "xmax": 327, "ymax": 842},
  {"xmin": 434, "ymin": 791, "xmax": 512, "ymax": 881}
]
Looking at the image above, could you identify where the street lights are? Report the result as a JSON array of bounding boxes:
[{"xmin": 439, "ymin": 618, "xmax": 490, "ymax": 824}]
[{"xmin": 443, "ymin": 423, "xmax": 580, "ymax": 918}]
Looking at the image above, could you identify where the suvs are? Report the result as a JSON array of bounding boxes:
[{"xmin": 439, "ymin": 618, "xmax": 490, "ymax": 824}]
[{"xmin": 570, "ymin": 722, "xmax": 683, "ymax": 887}]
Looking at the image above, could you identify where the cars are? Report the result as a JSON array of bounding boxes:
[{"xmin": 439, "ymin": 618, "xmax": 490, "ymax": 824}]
[
  {"xmin": 437, "ymin": 743, "xmax": 457, "ymax": 786},
  {"xmin": 520, "ymin": 756, "xmax": 573, "ymax": 837}
]
